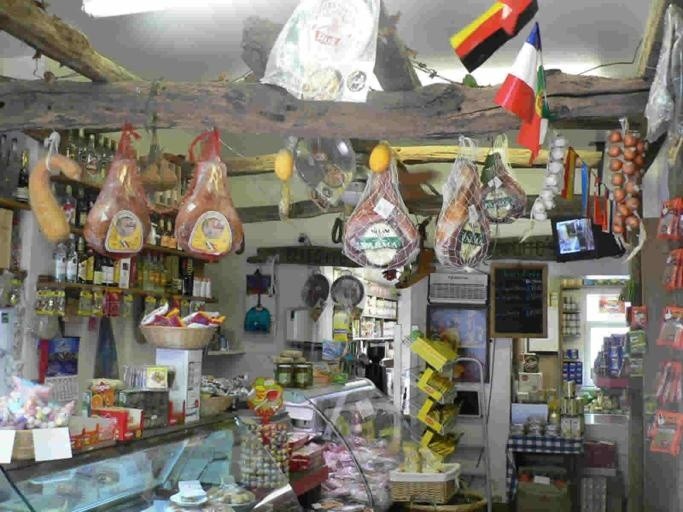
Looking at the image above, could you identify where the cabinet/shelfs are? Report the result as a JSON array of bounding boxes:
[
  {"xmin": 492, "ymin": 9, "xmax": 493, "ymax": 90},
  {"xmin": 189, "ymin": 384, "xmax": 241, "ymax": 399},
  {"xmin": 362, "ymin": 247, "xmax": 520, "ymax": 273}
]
[
  {"xmin": 0, "ymin": 125, "xmax": 220, "ymax": 306},
  {"xmin": 331, "ymin": 266, "xmax": 400, "ymax": 340},
  {"xmin": 430, "ymin": 390, "xmax": 493, "ymax": 512}
]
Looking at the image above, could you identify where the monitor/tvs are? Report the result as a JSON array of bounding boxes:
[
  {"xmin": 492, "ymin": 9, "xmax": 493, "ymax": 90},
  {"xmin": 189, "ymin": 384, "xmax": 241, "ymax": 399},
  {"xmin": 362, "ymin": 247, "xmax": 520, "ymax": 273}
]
[{"xmin": 548, "ymin": 215, "xmax": 620, "ymax": 262}]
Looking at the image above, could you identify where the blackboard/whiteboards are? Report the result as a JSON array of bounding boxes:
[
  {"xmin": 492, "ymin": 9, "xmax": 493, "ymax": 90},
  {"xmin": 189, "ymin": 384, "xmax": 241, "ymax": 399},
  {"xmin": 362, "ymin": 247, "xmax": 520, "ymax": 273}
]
[{"xmin": 489, "ymin": 261, "xmax": 549, "ymax": 340}]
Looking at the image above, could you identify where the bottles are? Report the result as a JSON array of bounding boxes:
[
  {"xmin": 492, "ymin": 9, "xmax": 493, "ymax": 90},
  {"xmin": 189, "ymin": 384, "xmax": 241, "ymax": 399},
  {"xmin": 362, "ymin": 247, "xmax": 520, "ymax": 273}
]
[
  {"xmin": 0, "ymin": 134, "xmax": 32, "ymax": 203},
  {"xmin": 54, "ymin": 128, "xmax": 184, "ymax": 249},
  {"xmin": 53, "ymin": 234, "xmax": 212, "ymax": 300}
]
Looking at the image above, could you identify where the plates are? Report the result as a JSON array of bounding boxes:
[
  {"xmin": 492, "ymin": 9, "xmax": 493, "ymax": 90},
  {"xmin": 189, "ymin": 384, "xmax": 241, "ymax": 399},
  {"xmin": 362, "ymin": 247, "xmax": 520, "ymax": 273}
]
[
  {"xmin": 168, "ymin": 491, "xmax": 210, "ymax": 508},
  {"xmin": 210, "ymin": 489, "xmax": 256, "ymax": 508}
]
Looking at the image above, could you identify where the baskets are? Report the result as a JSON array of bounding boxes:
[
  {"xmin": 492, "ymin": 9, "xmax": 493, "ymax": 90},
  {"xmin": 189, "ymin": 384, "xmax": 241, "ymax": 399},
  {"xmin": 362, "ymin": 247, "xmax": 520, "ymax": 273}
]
[
  {"xmin": 45, "ymin": 375, "xmax": 79, "ymax": 402},
  {"xmin": 390, "ymin": 462, "xmax": 461, "ymax": 504}
]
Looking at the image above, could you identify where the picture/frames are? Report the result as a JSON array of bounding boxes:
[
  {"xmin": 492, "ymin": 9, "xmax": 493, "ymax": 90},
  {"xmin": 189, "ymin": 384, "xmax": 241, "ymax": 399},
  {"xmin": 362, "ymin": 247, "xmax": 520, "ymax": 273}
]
[{"xmin": 425, "ymin": 302, "xmax": 491, "ymax": 383}]
